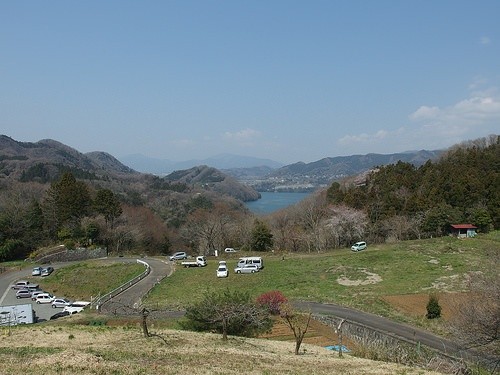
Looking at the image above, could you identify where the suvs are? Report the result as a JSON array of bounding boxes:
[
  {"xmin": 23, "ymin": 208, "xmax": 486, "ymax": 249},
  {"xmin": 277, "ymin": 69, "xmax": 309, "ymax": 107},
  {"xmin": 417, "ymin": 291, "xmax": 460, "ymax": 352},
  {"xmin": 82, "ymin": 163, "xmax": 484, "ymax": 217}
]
[
  {"xmin": 170, "ymin": 252, "xmax": 187, "ymax": 261},
  {"xmin": 225, "ymin": 247, "xmax": 236, "ymax": 253},
  {"xmin": 41, "ymin": 266, "xmax": 54, "ymax": 277},
  {"xmin": 234, "ymin": 264, "xmax": 259, "ymax": 274},
  {"xmin": 63, "ymin": 301, "xmax": 98, "ymax": 315},
  {"xmin": 15, "ymin": 288, "xmax": 33, "ymax": 299},
  {"xmin": 35, "ymin": 293, "xmax": 56, "ymax": 304}
]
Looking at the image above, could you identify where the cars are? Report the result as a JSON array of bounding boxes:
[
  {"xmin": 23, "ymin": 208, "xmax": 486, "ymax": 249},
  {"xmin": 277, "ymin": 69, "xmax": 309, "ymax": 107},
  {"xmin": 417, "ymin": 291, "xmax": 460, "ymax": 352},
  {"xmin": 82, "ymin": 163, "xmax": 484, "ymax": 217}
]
[
  {"xmin": 219, "ymin": 261, "xmax": 226, "ymax": 266},
  {"xmin": 32, "ymin": 267, "xmax": 42, "ymax": 276},
  {"xmin": 31, "ymin": 291, "xmax": 49, "ymax": 301},
  {"xmin": 216, "ymin": 265, "xmax": 229, "ymax": 277},
  {"xmin": 51, "ymin": 298, "xmax": 74, "ymax": 308}
]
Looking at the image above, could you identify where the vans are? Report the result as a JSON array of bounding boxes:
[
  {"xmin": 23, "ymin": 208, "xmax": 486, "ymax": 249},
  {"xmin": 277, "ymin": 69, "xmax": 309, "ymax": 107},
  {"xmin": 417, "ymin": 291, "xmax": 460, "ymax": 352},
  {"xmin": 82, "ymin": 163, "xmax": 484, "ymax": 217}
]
[
  {"xmin": 237, "ymin": 257, "xmax": 264, "ymax": 269},
  {"xmin": 351, "ymin": 242, "xmax": 367, "ymax": 252}
]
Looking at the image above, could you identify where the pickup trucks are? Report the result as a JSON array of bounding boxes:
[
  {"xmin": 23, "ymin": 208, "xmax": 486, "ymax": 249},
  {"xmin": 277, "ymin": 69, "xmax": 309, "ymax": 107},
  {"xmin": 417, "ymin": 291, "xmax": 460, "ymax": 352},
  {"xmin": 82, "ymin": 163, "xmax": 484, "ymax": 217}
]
[{"xmin": 11, "ymin": 281, "xmax": 39, "ymax": 291}]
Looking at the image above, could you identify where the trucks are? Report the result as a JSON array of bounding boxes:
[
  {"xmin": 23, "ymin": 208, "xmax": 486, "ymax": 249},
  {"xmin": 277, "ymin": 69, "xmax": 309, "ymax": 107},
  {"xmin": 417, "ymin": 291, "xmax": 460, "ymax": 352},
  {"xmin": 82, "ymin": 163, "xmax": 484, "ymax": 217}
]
[{"xmin": 182, "ymin": 256, "xmax": 207, "ymax": 268}]
